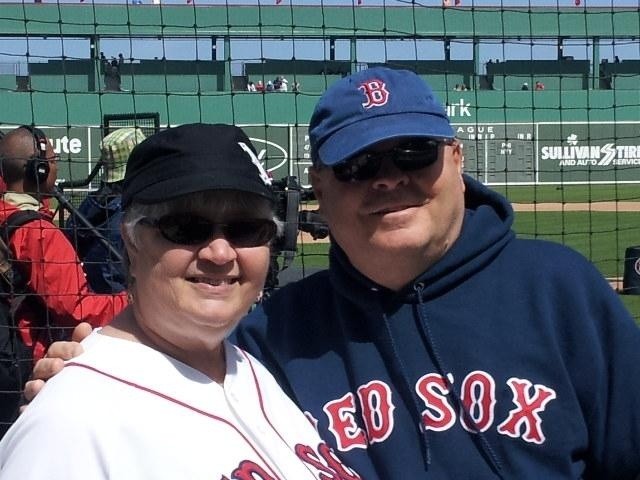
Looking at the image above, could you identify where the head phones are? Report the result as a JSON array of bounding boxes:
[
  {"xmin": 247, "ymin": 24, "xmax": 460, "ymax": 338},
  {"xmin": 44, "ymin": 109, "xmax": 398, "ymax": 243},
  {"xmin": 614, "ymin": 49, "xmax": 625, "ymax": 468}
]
[{"xmin": 21, "ymin": 125, "xmax": 50, "ymax": 182}]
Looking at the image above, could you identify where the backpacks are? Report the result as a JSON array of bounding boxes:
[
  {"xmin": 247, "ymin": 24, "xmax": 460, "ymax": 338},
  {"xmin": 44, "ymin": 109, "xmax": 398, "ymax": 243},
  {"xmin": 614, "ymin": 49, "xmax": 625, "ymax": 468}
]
[{"xmin": 0, "ymin": 210, "xmax": 53, "ymax": 439}]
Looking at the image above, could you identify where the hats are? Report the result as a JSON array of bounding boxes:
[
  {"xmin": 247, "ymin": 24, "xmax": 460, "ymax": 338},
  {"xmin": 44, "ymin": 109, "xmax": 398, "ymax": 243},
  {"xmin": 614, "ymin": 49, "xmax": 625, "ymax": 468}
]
[
  {"xmin": 308, "ymin": 66, "xmax": 457, "ymax": 172},
  {"xmin": 98, "ymin": 127, "xmax": 147, "ymax": 183},
  {"xmin": 121, "ymin": 123, "xmax": 274, "ymax": 209}
]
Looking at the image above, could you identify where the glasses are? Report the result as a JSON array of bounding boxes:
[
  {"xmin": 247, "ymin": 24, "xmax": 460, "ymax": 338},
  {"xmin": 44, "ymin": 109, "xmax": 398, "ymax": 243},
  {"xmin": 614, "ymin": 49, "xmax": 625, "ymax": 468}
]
[
  {"xmin": 320, "ymin": 138, "xmax": 453, "ymax": 182},
  {"xmin": 140, "ymin": 212, "xmax": 277, "ymax": 247}
]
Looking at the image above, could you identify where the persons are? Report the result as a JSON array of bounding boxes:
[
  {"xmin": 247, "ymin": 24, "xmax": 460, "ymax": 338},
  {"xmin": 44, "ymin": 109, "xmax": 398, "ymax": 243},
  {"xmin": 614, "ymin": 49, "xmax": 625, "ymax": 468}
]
[
  {"xmin": 2, "ymin": 125, "xmax": 131, "ymax": 430},
  {"xmin": 1, "ymin": 124, "xmax": 371, "ymax": 479},
  {"xmin": 454, "ymin": 84, "xmax": 458, "ymax": 90},
  {"xmin": 56, "ymin": 125, "xmax": 145, "ymax": 296},
  {"xmin": 247, "ymin": 75, "xmax": 289, "ymax": 91},
  {"xmin": 19, "ymin": 67, "xmax": 640, "ymax": 478},
  {"xmin": 467, "ymin": 86, "xmax": 473, "ymax": 91},
  {"xmin": 456, "ymin": 85, "xmax": 467, "ymax": 92},
  {"xmin": 521, "ymin": 83, "xmax": 528, "ymax": 90},
  {"xmin": 535, "ymin": 81, "xmax": 544, "ymax": 90},
  {"xmin": 119, "ymin": 53, "xmax": 124, "ymax": 72},
  {"xmin": 101, "ymin": 52, "xmax": 106, "ymax": 61},
  {"xmin": 104, "ymin": 61, "xmax": 122, "ymax": 91}
]
